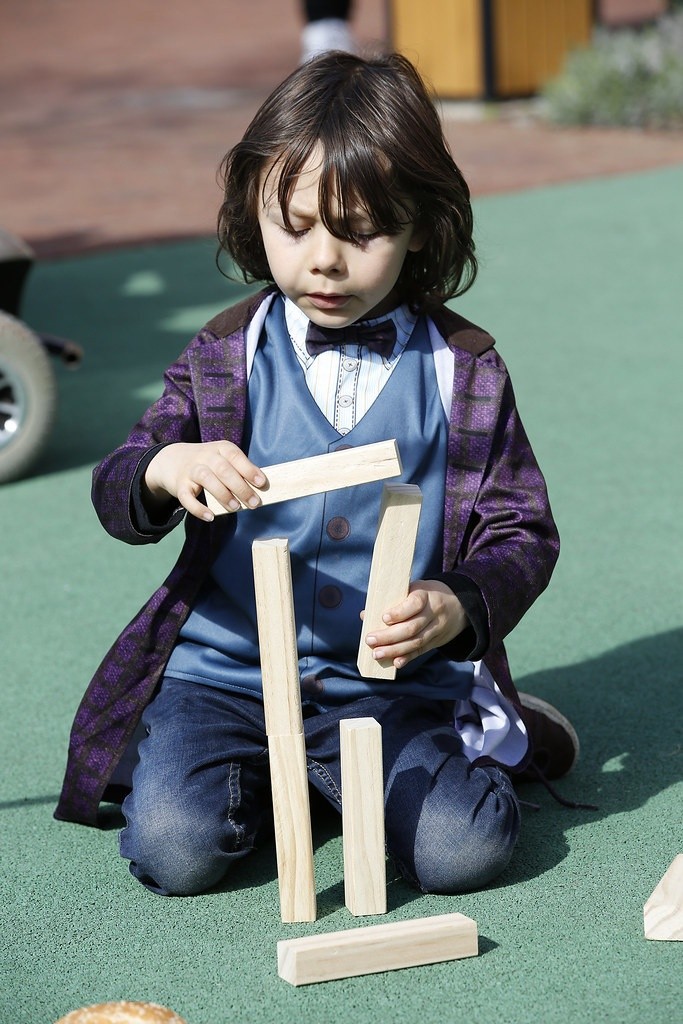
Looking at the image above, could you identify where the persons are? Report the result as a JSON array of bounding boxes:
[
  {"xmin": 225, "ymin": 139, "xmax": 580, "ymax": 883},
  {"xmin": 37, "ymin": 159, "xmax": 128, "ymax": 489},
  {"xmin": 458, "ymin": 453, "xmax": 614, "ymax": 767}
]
[{"xmin": 52, "ymin": 50, "xmax": 581, "ymax": 897}]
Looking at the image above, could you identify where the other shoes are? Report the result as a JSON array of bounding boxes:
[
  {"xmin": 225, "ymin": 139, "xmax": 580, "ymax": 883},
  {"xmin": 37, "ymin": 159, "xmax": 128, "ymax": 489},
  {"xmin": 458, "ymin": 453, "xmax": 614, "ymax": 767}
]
[{"xmin": 515, "ymin": 689, "xmax": 600, "ymax": 812}]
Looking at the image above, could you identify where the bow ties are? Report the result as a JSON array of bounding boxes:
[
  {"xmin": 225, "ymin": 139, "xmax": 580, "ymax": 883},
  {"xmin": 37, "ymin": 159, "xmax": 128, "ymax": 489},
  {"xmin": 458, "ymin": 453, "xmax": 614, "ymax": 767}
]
[{"xmin": 305, "ymin": 318, "xmax": 398, "ymax": 359}]
